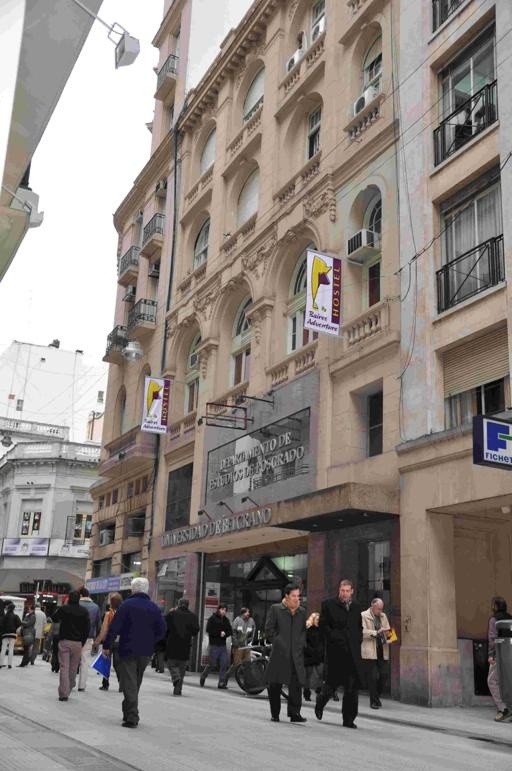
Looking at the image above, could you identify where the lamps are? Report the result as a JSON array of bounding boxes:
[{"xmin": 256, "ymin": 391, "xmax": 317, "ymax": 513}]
[
  {"xmin": 1, "ymin": 184, "xmax": 44, "ymax": 227},
  {"xmin": 198, "ymin": 396, "xmax": 274, "ymax": 430},
  {"xmin": 198, "ymin": 496, "xmax": 260, "ymax": 521},
  {"xmin": 74, "ymin": 0, "xmax": 140, "ymax": 69}
]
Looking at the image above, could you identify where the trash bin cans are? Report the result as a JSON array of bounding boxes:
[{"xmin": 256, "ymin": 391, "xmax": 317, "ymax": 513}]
[{"xmin": 494, "ymin": 619, "xmax": 512, "ymax": 723}]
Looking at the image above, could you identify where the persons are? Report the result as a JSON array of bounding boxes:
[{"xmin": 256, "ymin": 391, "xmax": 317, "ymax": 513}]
[
  {"xmin": 15, "ymin": 602, "xmax": 46, "ymax": 668},
  {"xmin": 94, "ymin": 591, "xmax": 124, "ymax": 693},
  {"xmin": 151, "ymin": 598, "xmax": 200, "ymax": 696},
  {"xmin": 315, "ymin": 580, "xmax": 363, "ymax": 729},
  {"xmin": 1, "ymin": 600, "xmax": 22, "ymax": 668},
  {"xmin": 224, "ymin": 607, "xmax": 256, "ymax": 685},
  {"xmin": 42, "ymin": 587, "xmax": 101, "ymax": 703},
  {"xmin": 304, "ymin": 612, "xmax": 340, "ymax": 702},
  {"xmin": 360, "ymin": 598, "xmax": 389, "ymax": 708},
  {"xmin": 486, "ymin": 597, "xmax": 512, "ymax": 723},
  {"xmin": 199, "ymin": 603, "xmax": 233, "ymax": 689},
  {"xmin": 265, "ymin": 583, "xmax": 309, "ymax": 723},
  {"xmin": 102, "ymin": 578, "xmax": 167, "ymax": 727}
]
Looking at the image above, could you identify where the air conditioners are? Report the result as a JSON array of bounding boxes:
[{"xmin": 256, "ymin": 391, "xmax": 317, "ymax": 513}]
[
  {"xmin": 285, "ymin": 49, "xmax": 302, "ymax": 72},
  {"xmin": 346, "ymin": 228, "xmax": 380, "ymax": 263},
  {"xmin": 311, "ymin": 17, "xmax": 325, "ymax": 45},
  {"xmin": 99, "ymin": 530, "xmax": 113, "ymax": 546},
  {"xmin": 354, "ymin": 86, "xmax": 375, "ymax": 117},
  {"xmin": 188, "ymin": 352, "xmax": 199, "ymax": 370}
]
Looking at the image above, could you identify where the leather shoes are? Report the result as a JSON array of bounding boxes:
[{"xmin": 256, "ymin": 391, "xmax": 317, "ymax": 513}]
[
  {"xmin": 270, "ymin": 714, "xmax": 280, "ymax": 722},
  {"xmin": 370, "ymin": 699, "xmax": 382, "ymax": 709},
  {"xmin": 290, "ymin": 712, "xmax": 307, "ymax": 722},
  {"xmin": 304, "ymin": 696, "xmax": 312, "ymax": 703},
  {"xmin": 315, "ymin": 694, "xmax": 324, "ymax": 720},
  {"xmin": 121, "ymin": 721, "xmax": 139, "ymax": 728},
  {"xmin": 342, "ymin": 720, "xmax": 357, "ymax": 729}
]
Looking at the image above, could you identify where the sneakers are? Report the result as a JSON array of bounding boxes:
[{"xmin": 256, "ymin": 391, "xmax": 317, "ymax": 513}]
[
  {"xmin": 173, "ymin": 679, "xmax": 183, "ymax": 693},
  {"xmin": 200, "ymin": 675, "xmax": 206, "ymax": 688},
  {"xmin": 58, "ymin": 696, "xmax": 69, "ymax": 703},
  {"xmin": 494, "ymin": 709, "xmax": 512, "ymax": 723},
  {"xmin": 218, "ymin": 684, "xmax": 229, "ymax": 690},
  {"xmin": 0, "ymin": 655, "xmax": 59, "ymax": 674},
  {"xmin": 99, "ymin": 685, "xmax": 109, "ymax": 692},
  {"xmin": 77, "ymin": 687, "xmax": 88, "ymax": 694}
]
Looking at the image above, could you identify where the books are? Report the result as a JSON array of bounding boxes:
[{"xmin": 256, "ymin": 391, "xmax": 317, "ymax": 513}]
[{"xmin": 379, "ymin": 629, "xmax": 397, "ymax": 645}]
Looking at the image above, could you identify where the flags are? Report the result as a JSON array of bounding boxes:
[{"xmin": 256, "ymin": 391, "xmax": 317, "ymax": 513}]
[
  {"xmin": 304, "ymin": 250, "xmax": 341, "ymax": 338},
  {"xmin": 140, "ymin": 376, "xmax": 171, "ymax": 434}
]
